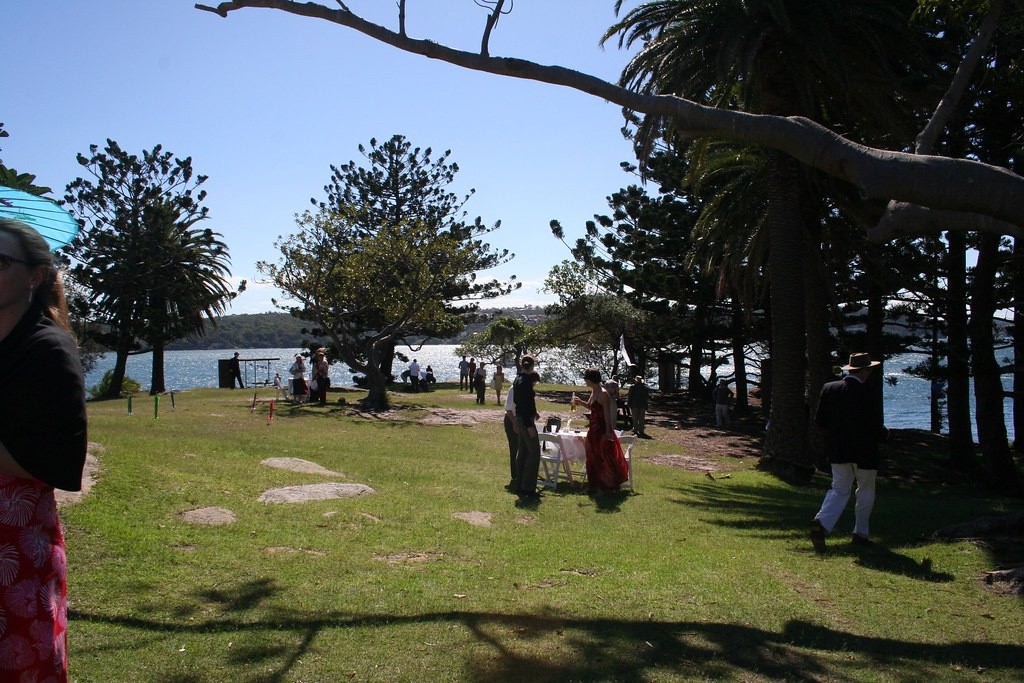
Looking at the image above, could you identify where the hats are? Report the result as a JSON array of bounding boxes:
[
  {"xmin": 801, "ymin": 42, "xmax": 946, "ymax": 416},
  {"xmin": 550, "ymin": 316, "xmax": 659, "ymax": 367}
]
[{"xmin": 841, "ymin": 352, "xmax": 881, "ymax": 370}]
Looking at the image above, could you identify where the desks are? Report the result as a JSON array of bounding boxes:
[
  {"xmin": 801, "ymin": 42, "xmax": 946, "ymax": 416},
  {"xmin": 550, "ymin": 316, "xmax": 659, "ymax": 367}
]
[
  {"xmin": 540, "ymin": 431, "xmax": 589, "ymax": 488},
  {"xmin": 287, "ymin": 378, "xmax": 310, "ymax": 404}
]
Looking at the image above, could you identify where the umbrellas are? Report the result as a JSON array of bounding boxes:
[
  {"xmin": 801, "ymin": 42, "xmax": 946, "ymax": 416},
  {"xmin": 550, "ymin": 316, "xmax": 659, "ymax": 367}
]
[{"xmin": 0, "ymin": 186, "xmax": 79, "ymax": 253}]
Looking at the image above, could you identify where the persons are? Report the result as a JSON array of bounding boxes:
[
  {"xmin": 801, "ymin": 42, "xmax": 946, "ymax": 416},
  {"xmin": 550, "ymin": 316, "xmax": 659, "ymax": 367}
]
[
  {"xmin": 513, "ymin": 356, "xmax": 541, "ymax": 498},
  {"xmin": 811, "ymin": 352, "xmax": 897, "ymax": 553},
  {"xmin": 289, "ymin": 348, "xmax": 328, "ymax": 405},
  {"xmin": 468, "ymin": 358, "xmax": 476, "ymax": 393},
  {"xmin": 425, "ymin": 365, "xmax": 436, "ymax": 383},
  {"xmin": 474, "ymin": 362, "xmax": 486, "ymax": 405},
  {"xmin": 712, "ymin": 379, "xmax": 734, "ymax": 428},
  {"xmin": 264, "ymin": 380, "xmax": 271, "ymax": 386},
  {"xmin": 229, "ymin": 352, "xmax": 246, "ymax": 389},
  {"xmin": 504, "ymin": 371, "xmax": 541, "ymax": 491},
  {"xmin": 627, "ymin": 376, "xmax": 649, "ymax": 436},
  {"xmin": 459, "ymin": 356, "xmax": 469, "ymax": 391},
  {"xmin": 401, "ymin": 370, "xmax": 410, "ymax": 384},
  {"xmin": 0, "ymin": 219, "xmax": 87, "ymax": 683},
  {"xmin": 409, "ymin": 359, "xmax": 420, "ymax": 391},
  {"xmin": 604, "ymin": 375, "xmax": 621, "ymax": 428},
  {"xmin": 569, "ymin": 368, "xmax": 628, "ymax": 494},
  {"xmin": 272, "ymin": 374, "xmax": 281, "ymax": 386},
  {"xmin": 493, "ymin": 365, "xmax": 504, "ymax": 406}
]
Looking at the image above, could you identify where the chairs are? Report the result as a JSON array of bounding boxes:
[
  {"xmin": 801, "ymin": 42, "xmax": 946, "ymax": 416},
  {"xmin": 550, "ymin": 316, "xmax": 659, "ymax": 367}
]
[{"xmin": 535, "ymin": 423, "xmax": 637, "ymax": 490}]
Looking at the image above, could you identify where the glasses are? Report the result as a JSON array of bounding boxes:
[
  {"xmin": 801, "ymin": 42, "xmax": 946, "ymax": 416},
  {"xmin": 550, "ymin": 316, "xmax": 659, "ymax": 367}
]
[{"xmin": 0, "ymin": 254, "xmax": 28, "ymax": 270}]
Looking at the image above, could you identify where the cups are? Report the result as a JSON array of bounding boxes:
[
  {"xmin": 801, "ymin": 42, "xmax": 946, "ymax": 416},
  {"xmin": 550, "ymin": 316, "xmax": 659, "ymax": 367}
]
[{"xmin": 551, "ymin": 425, "xmax": 557, "ymax": 434}]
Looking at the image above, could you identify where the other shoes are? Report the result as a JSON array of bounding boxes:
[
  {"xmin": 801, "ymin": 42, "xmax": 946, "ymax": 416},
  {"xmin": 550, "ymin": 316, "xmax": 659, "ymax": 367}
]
[
  {"xmin": 851, "ymin": 533, "xmax": 875, "ymax": 547},
  {"xmin": 810, "ymin": 519, "xmax": 826, "ymax": 552}
]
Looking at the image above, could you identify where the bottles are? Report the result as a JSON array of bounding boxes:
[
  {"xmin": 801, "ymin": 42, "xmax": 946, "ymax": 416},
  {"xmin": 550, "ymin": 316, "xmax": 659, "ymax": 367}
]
[{"xmin": 570, "ymin": 391, "xmax": 576, "ymax": 412}]
